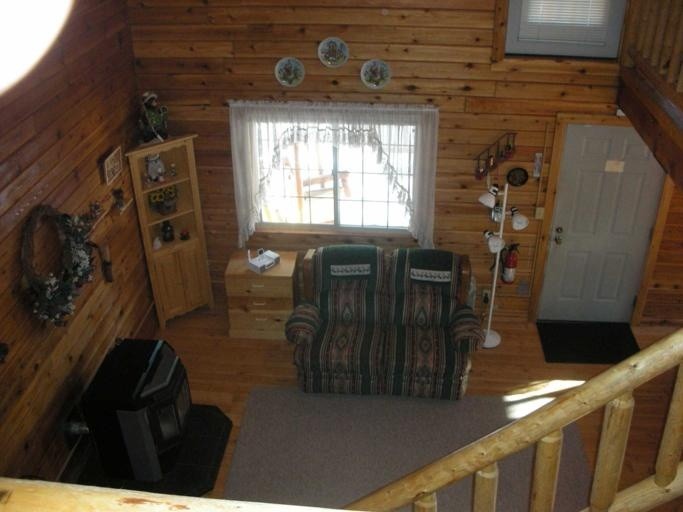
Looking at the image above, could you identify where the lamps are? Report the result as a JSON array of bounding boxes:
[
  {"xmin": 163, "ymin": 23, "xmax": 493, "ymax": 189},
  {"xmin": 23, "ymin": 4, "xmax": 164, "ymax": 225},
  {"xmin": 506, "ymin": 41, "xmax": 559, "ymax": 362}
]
[{"xmin": 478, "ymin": 184, "xmax": 529, "ymax": 349}]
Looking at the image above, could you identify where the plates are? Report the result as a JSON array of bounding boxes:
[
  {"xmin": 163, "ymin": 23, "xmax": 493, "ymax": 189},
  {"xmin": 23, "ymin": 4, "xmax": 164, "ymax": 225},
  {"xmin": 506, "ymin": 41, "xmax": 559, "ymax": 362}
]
[
  {"xmin": 274, "ymin": 57, "xmax": 305, "ymax": 88},
  {"xmin": 317, "ymin": 37, "xmax": 348, "ymax": 69},
  {"xmin": 359, "ymin": 59, "xmax": 390, "ymax": 90}
]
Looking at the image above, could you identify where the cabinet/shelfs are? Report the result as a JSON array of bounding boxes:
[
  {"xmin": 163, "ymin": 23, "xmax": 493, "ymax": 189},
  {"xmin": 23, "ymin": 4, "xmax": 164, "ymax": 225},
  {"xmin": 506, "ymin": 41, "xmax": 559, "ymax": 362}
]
[
  {"xmin": 124, "ymin": 133, "xmax": 217, "ymax": 330},
  {"xmin": 224, "ymin": 250, "xmax": 299, "ymax": 340}
]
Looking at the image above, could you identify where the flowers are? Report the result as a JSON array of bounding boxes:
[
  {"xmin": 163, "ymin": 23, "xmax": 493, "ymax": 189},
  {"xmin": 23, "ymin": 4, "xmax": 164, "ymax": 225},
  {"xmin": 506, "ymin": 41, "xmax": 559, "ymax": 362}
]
[
  {"xmin": 149, "ymin": 184, "xmax": 178, "ymax": 203},
  {"xmin": 16, "ymin": 202, "xmax": 97, "ymax": 327}
]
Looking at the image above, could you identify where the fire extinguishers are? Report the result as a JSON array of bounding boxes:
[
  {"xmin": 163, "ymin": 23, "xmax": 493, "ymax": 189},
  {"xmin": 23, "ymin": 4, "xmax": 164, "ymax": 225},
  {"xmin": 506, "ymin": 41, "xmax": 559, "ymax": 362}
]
[{"xmin": 489, "ymin": 244, "xmax": 519, "ymax": 284}]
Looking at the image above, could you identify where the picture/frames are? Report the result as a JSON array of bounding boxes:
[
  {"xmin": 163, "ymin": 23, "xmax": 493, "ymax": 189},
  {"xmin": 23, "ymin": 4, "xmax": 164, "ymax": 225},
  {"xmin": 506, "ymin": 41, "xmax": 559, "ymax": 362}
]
[{"xmin": 102, "ymin": 145, "xmax": 123, "ymax": 186}]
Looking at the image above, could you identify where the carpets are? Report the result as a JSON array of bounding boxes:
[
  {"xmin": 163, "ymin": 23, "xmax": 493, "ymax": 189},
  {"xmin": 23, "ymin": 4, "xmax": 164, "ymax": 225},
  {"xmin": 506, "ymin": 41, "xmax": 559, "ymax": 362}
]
[
  {"xmin": 536, "ymin": 319, "xmax": 641, "ymax": 364},
  {"xmin": 223, "ymin": 389, "xmax": 593, "ymax": 512}
]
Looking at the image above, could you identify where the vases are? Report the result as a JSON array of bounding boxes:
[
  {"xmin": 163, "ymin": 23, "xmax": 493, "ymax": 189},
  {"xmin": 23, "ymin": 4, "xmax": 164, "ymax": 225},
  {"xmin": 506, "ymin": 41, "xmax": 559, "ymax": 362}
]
[{"xmin": 157, "ymin": 199, "xmax": 177, "ymax": 215}]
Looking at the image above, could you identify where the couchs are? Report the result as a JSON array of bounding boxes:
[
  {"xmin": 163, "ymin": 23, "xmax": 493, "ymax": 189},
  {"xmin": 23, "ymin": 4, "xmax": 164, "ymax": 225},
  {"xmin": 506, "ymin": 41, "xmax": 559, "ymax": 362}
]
[{"xmin": 285, "ymin": 244, "xmax": 486, "ymax": 401}]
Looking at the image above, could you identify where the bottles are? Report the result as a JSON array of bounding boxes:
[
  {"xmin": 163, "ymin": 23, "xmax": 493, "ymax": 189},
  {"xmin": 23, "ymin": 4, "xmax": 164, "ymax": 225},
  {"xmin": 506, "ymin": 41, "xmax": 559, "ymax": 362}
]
[{"xmin": 161, "ymin": 222, "xmax": 174, "ymax": 241}]
